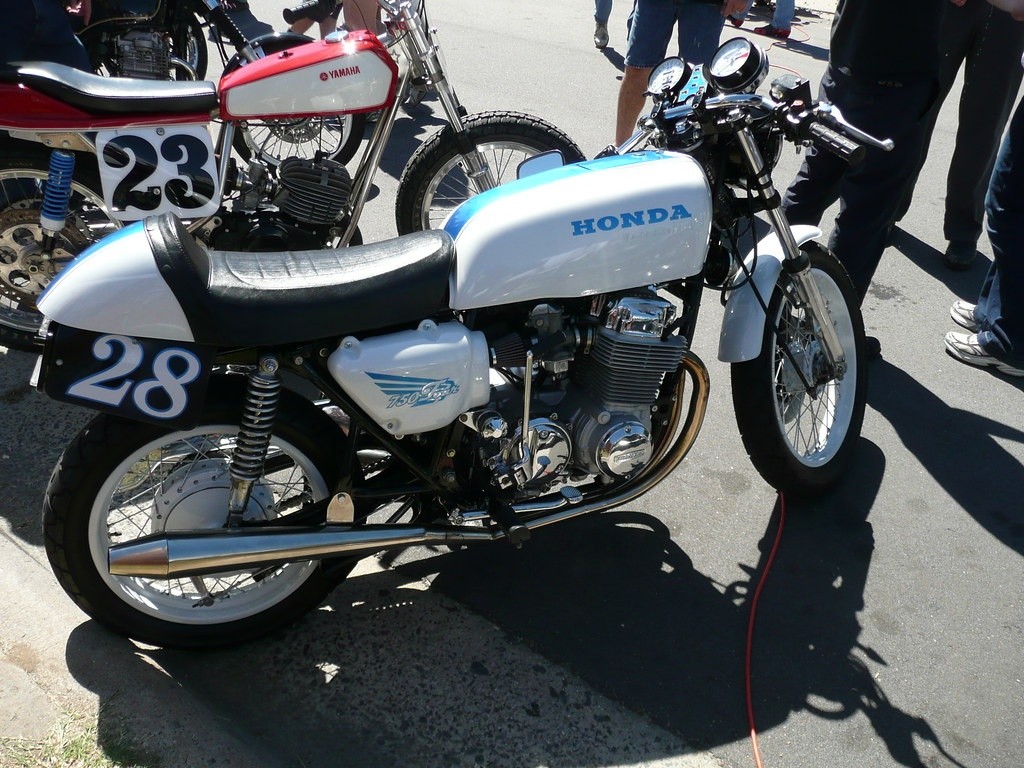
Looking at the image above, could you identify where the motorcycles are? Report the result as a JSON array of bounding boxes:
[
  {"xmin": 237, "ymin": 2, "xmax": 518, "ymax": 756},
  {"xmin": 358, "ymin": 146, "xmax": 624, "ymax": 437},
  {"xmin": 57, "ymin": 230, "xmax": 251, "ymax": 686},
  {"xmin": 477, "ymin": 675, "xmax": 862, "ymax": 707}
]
[
  {"xmin": 29, "ymin": 36, "xmax": 895, "ymax": 653},
  {"xmin": 0, "ymin": 1, "xmax": 590, "ymax": 354}
]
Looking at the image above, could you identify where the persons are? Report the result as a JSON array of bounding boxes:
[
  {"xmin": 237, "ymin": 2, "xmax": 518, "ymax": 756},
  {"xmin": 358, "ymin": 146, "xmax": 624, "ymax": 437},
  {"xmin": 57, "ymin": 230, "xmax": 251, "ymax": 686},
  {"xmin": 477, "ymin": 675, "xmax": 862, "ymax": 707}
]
[
  {"xmin": 593, "ymin": 0, "xmax": 794, "ymax": 161},
  {"xmin": 775, "ymin": 0, "xmax": 1024, "ymax": 377},
  {"xmin": 286, "ymin": 0, "xmax": 381, "ymax": 53}
]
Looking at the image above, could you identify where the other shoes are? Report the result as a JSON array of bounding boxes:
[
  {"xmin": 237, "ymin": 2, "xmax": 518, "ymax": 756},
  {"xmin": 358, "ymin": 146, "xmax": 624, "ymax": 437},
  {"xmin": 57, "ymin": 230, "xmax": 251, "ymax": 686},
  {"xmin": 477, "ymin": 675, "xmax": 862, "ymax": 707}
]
[
  {"xmin": 727, "ymin": 14, "xmax": 744, "ymax": 28},
  {"xmin": 944, "ymin": 240, "xmax": 976, "ymax": 267},
  {"xmin": 754, "ymin": 25, "xmax": 791, "ymax": 38},
  {"xmin": 865, "ymin": 337, "xmax": 881, "ymax": 360},
  {"xmin": 884, "ymin": 223, "xmax": 895, "ymax": 247},
  {"xmin": 594, "ymin": 22, "xmax": 609, "ymax": 48}
]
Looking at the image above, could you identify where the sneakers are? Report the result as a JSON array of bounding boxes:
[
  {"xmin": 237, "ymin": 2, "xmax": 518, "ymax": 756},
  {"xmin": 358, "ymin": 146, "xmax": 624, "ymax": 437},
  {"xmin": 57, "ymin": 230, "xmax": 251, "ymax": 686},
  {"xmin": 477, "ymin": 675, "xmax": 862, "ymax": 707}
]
[
  {"xmin": 944, "ymin": 331, "xmax": 1024, "ymax": 376},
  {"xmin": 950, "ymin": 300, "xmax": 977, "ymax": 333}
]
